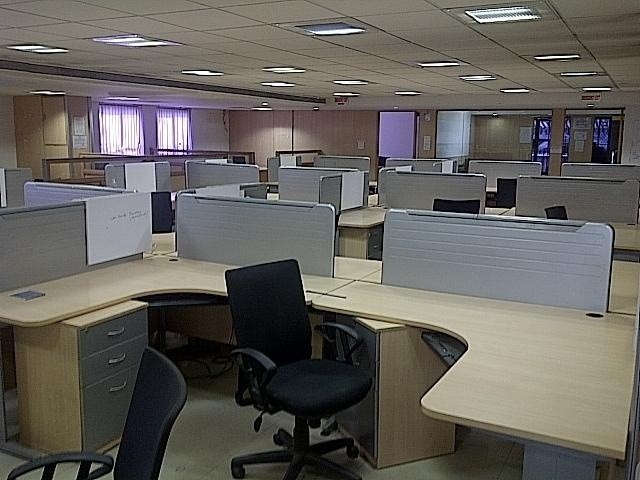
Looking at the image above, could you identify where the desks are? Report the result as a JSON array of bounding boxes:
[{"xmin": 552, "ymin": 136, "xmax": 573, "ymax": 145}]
[{"xmin": 0, "ymin": 151, "xmax": 639, "ymax": 480}]
